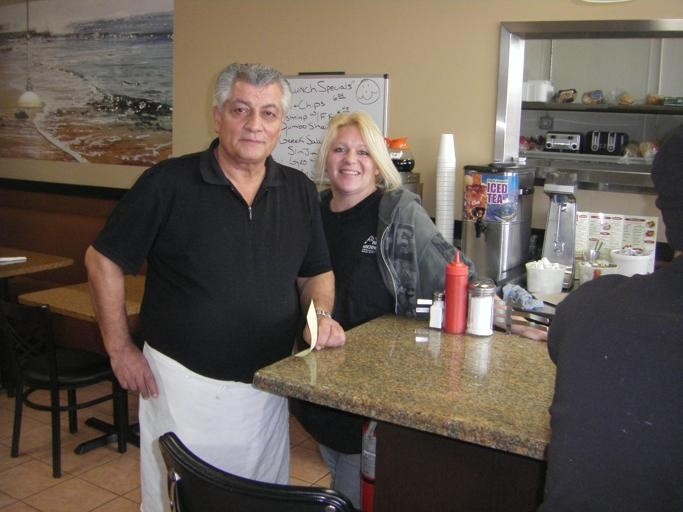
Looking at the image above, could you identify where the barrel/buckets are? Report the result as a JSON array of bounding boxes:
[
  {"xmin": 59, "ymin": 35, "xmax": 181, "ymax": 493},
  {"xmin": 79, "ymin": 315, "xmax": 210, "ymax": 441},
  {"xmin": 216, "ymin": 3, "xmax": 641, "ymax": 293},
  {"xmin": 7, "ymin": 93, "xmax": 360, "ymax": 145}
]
[{"xmin": 461, "ymin": 162, "xmax": 536, "ymax": 282}]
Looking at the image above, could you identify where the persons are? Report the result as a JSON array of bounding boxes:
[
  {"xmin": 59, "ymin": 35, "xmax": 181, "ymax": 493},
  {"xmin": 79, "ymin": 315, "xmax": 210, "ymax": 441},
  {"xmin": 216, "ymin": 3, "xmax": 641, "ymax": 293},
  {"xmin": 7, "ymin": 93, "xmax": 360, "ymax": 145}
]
[
  {"xmin": 82, "ymin": 62, "xmax": 345, "ymax": 512},
  {"xmin": 536, "ymin": 119, "xmax": 683, "ymax": 512},
  {"xmin": 287, "ymin": 112, "xmax": 549, "ymax": 512}
]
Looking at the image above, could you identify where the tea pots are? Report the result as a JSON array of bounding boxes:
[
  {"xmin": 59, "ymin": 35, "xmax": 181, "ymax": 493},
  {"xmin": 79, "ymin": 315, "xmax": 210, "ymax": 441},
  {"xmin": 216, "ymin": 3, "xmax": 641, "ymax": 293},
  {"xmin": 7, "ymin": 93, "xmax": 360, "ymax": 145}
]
[{"xmin": 383, "ymin": 137, "xmax": 415, "ymax": 172}]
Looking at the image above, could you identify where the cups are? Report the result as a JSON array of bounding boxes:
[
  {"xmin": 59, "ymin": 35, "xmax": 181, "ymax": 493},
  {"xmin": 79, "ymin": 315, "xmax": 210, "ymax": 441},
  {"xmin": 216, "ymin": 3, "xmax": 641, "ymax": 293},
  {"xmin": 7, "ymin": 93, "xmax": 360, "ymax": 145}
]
[
  {"xmin": 525, "ymin": 260, "xmax": 566, "ymax": 296},
  {"xmin": 575, "ymin": 248, "xmax": 652, "ymax": 283},
  {"xmin": 435, "ymin": 133, "xmax": 458, "ymax": 246}
]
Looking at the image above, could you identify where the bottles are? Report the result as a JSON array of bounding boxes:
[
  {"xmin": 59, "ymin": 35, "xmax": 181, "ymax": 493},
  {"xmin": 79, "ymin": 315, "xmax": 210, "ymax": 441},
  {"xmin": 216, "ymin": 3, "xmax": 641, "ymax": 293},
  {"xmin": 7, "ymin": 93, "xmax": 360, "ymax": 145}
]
[{"xmin": 428, "ymin": 252, "xmax": 497, "ymax": 338}]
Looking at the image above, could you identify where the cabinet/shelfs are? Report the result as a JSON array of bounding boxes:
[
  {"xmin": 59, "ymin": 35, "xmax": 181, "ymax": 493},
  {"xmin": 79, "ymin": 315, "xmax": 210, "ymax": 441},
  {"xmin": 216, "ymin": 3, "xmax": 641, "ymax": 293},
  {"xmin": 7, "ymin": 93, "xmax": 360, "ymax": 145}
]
[{"xmin": 516, "ymin": 98, "xmax": 681, "ymax": 190}]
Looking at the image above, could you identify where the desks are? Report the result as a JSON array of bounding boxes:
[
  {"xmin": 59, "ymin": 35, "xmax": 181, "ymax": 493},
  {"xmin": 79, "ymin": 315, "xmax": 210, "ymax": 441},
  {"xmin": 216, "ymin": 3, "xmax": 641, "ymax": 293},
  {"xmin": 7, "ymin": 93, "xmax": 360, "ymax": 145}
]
[{"xmin": 1, "ymin": 244, "xmax": 149, "ymax": 456}]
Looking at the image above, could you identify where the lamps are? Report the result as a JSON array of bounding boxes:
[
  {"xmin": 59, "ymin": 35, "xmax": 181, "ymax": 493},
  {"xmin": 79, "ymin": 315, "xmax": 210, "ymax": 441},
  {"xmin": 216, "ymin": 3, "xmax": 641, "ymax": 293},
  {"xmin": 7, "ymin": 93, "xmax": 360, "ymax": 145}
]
[{"xmin": 16, "ymin": 1, "xmax": 43, "ymax": 121}]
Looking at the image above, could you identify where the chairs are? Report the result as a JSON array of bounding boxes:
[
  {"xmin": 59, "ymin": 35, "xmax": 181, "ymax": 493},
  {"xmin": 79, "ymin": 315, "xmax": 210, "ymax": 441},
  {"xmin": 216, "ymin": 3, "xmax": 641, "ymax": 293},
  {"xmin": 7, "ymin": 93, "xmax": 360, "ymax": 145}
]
[
  {"xmin": 158, "ymin": 432, "xmax": 356, "ymax": 511},
  {"xmin": 2, "ymin": 303, "xmax": 128, "ymax": 478}
]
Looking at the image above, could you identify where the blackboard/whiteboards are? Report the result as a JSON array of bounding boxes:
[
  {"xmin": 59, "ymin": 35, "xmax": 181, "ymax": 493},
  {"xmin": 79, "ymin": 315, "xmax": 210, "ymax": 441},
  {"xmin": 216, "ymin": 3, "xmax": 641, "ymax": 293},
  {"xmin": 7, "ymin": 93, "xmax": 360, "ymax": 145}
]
[{"xmin": 270, "ymin": 73, "xmax": 387, "ymax": 184}]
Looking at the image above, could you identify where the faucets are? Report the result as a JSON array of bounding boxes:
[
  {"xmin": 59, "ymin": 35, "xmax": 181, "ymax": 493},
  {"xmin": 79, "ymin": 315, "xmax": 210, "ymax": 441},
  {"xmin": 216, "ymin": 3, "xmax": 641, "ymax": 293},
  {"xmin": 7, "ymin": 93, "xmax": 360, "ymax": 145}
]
[{"xmin": 471, "ymin": 206, "xmax": 487, "ymax": 237}]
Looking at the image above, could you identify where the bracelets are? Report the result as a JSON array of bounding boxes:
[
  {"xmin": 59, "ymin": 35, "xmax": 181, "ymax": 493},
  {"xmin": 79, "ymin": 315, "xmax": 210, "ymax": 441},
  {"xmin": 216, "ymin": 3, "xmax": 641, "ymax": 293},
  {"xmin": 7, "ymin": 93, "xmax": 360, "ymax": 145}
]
[{"xmin": 314, "ymin": 310, "xmax": 335, "ymax": 319}]
